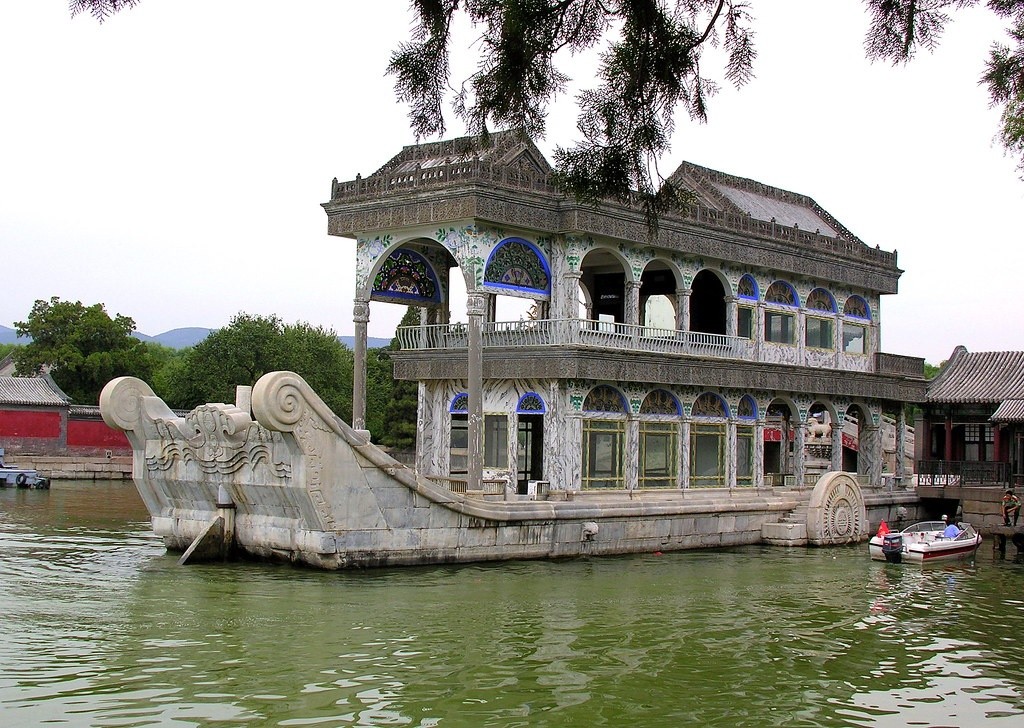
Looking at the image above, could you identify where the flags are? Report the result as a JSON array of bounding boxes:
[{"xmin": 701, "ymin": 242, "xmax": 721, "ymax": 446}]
[{"xmin": 877, "ymin": 518, "xmax": 889, "ymax": 538}]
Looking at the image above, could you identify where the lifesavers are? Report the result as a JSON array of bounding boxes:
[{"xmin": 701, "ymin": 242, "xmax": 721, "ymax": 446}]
[{"xmin": 16, "ymin": 473, "xmax": 27, "ymax": 486}]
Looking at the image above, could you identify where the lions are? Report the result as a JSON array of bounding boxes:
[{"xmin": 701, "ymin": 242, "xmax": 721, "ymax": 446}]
[{"xmin": 806, "ymin": 418, "xmax": 832, "ymax": 438}]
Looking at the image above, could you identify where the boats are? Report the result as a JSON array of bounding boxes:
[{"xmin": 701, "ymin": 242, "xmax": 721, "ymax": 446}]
[
  {"xmin": 868, "ymin": 514, "xmax": 983, "ymax": 563},
  {"xmin": 0, "ymin": 447, "xmax": 50, "ymax": 489}
]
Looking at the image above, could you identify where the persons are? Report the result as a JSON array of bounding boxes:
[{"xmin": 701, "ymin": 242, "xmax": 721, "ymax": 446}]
[
  {"xmin": 1001, "ymin": 490, "xmax": 1022, "ymax": 527},
  {"xmin": 944, "ymin": 519, "xmax": 963, "ymax": 541}
]
